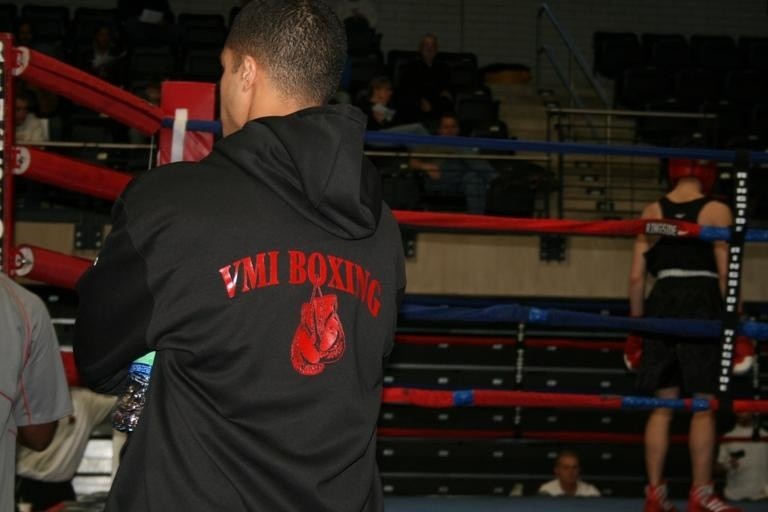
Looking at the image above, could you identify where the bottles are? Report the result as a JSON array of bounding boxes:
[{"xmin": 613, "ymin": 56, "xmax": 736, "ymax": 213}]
[{"xmin": 111, "ymin": 347, "xmax": 155, "ymax": 432}]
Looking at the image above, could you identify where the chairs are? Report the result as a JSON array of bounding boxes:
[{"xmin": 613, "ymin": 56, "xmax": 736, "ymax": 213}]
[{"xmin": 0, "ymin": 1, "xmax": 766, "ymax": 495}]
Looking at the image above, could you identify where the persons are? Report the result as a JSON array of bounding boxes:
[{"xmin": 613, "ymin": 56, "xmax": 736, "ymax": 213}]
[
  {"xmin": 1, "ymin": 2, "xmax": 768, "ymax": 234},
  {"xmin": 70, "ymin": 3, "xmax": 406, "ymax": 510},
  {"xmin": 624, "ymin": 156, "xmax": 755, "ymax": 512},
  {"xmin": 0, "ymin": 273, "xmax": 114, "ymax": 512},
  {"xmin": 714, "ymin": 411, "xmax": 768, "ymax": 512},
  {"xmin": 539, "ymin": 453, "xmax": 601, "ymax": 497}
]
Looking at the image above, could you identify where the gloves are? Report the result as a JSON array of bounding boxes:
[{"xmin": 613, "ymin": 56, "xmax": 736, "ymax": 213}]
[
  {"xmin": 730, "ymin": 336, "xmax": 757, "ymax": 375},
  {"xmin": 624, "ymin": 331, "xmax": 644, "ymax": 373}
]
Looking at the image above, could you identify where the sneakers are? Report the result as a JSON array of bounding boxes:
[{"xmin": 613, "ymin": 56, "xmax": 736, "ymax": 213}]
[
  {"xmin": 645, "ymin": 482, "xmax": 681, "ymax": 512},
  {"xmin": 688, "ymin": 481, "xmax": 742, "ymax": 512}
]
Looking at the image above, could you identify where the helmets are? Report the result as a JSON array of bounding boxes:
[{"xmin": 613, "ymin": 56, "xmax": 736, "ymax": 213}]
[{"xmin": 666, "ymin": 154, "xmax": 716, "ymax": 194}]
